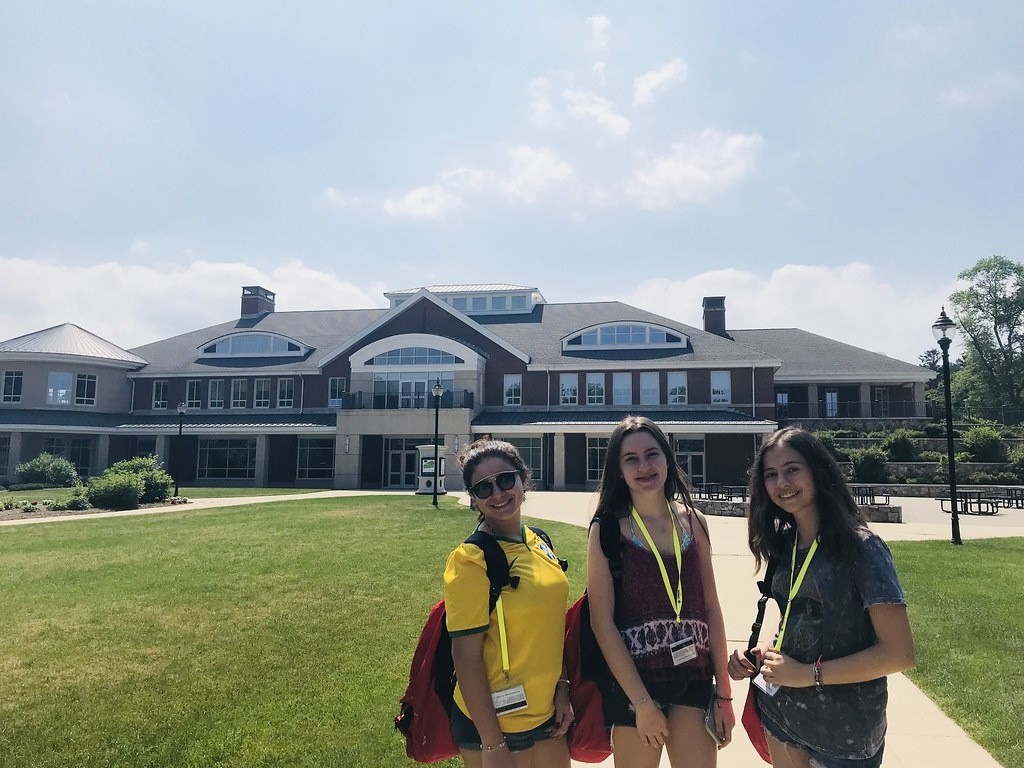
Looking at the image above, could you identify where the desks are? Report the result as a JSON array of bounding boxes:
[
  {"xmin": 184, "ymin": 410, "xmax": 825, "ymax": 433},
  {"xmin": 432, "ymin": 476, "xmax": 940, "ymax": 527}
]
[
  {"xmin": 1005, "ymin": 488, "xmax": 1024, "ymax": 508},
  {"xmin": 850, "ymin": 486, "xmax": 876, "ymax": 505},
  {"xmin": 722, "ymin": 486, "xmax": 749, "ymax": 502},
  {"xmin": 946, "ymin": 489, "xmax": 985, "ymax": 515},
  {"xmin": 696, "ymin": 482, "xmax": 722, "ymax": 500}
]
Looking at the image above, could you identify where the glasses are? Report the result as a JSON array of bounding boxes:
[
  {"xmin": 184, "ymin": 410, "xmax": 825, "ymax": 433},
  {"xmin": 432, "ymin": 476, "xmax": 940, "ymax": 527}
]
[{"xmin": 468, "ymin": 469, "xmax": 520, "ymax": 499}]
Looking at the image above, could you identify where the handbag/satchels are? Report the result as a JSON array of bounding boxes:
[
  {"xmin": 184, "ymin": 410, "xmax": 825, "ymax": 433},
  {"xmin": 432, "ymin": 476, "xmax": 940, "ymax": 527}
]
[{"xmin": 741, "ymin": 685, "xmax": 773, "ymax": 766}]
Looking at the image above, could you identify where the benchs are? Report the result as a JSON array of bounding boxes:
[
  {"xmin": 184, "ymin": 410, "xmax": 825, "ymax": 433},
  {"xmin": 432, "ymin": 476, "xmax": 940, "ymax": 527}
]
[
  {"xmin": 935, "ymin": 496, "xmax": 1024, "ymax": 515},
  {"xmin": 691, "ymin": 489, "xmax": 751, "ymax": 502},
  {"xmin": 858, "ymin": 492, "xmax": 890, "ymax": 505}
]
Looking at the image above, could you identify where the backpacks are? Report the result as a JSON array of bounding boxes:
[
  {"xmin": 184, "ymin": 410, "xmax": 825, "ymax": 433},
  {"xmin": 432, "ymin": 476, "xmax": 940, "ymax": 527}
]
[
  {"xmin": 393, "ymin": 526, "xmax": 553, "ymax": 763},
  {"xmin": 564, "ymin": 515, "xmax": 619, "ymax": 763}
]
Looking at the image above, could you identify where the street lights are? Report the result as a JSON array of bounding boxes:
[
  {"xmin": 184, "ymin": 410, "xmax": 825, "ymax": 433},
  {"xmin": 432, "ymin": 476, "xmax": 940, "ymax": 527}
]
[
  {"xmin": 171, "ymin": 398, "xmax": 188, "ymax": 497},
  {"xmin": 930, "ymin": 304, "xmax": 963, "ymax": 547},
  {"xmin": 431, "ymin": 377, "xmax": 445, "ymax": 506}
]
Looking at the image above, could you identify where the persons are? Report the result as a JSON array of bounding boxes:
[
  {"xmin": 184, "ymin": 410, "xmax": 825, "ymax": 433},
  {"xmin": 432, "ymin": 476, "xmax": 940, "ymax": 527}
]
[
  {"xmin": 585, "ymin": 416, "xmax": 736, "ymax": 768},
  {"xmin": 443, "ymin": 435, "xmax": 575, "ymax": 768},
  {"xmin": 727, "ymin": 426, "xmax": 915, "ymax": 768}
]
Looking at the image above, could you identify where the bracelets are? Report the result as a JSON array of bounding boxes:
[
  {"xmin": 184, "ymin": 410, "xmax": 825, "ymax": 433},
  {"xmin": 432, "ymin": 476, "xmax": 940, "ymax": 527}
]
[
  {"xmin": 633, "ymin": 694, "xmax": 652, "ymax": 710},
  {"xmin": 558, "ymin": 679, "xmax": 570, "ymax": 686},
  {"xmin": 480, "ymin": 736, "xmax": 507, "ymax": 752},
  {"xmin": 813, "ymin": 654, "xmax": 826, "ymax": 691},
  {"xmin": 715, "ymin": 694, "xmax": 733, "ymax": 708}
]
